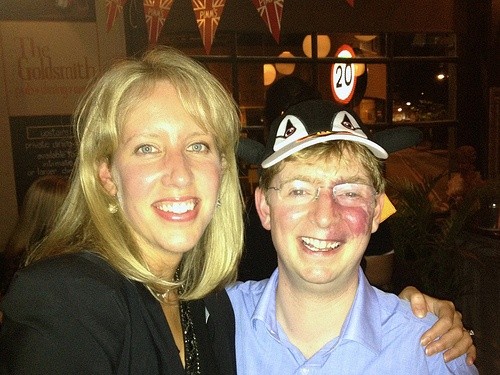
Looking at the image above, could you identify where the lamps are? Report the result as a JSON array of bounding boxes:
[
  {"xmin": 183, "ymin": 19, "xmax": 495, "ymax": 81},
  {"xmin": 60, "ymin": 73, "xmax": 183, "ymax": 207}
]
[{"xmin": 264, "ymin": 34, "xmax": 331, "ymax": 86}]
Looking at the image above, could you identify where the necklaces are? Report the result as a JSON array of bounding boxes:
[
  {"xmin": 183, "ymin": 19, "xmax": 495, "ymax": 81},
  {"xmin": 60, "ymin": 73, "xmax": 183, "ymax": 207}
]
[{"xmin": 462, "ymin": 329, "xmax": 476, "ymax": 336}]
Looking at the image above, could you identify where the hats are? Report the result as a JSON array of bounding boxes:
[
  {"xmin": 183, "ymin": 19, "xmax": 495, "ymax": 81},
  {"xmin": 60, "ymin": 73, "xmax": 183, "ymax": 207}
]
[{"xmin": 260, "ymin": 99, "xmax": 388, "ymax": 169}]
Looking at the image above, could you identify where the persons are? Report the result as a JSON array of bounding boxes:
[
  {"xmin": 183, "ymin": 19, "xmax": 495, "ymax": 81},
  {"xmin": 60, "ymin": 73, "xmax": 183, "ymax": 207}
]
[
  {"xmin": 0, "ymin": 174, "xmax": 70, "ymax": 299},
  {"xmin": 225, "ymin": 96, "xmax": 480, "ymax": 375},
  {"xmin": 238, "ymin": 77, "xmax": 396, "ymax": 286},
  {"xmin": 0, "ymin": 46, "xmax": 475, "ymax": 375}
]
